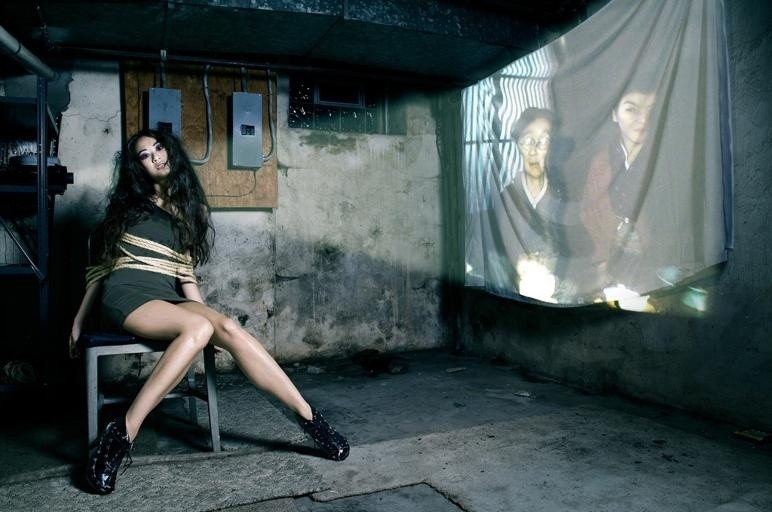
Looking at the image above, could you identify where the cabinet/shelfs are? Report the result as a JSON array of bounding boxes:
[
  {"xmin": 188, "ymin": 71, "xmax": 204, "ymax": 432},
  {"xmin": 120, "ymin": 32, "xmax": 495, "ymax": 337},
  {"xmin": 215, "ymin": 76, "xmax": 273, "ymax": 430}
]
[{"xmin": 0, "ymin": 72, "xmax": 54, "ymax": 393}]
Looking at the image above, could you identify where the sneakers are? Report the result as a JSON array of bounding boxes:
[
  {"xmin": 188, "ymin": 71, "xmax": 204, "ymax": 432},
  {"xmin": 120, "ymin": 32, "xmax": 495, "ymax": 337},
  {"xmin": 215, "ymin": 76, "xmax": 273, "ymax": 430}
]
[
  {"xmin": 85, "ymin": 419, "xmax": 133, "ymax": 496},
  {"xmin": 295, "ymin": 402, "xmax": 351, "ymax": 462}
]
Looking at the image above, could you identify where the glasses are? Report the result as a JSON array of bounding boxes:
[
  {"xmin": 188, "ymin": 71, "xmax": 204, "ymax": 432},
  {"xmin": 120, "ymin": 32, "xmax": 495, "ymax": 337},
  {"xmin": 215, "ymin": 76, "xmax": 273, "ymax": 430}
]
[{"xmin": 514, "ymin": 135, "xmax": 551, "ymax": 149}]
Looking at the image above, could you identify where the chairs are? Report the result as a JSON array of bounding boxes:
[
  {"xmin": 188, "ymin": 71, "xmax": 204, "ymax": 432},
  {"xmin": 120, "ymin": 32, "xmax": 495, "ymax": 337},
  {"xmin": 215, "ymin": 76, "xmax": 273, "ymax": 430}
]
[{"xmin": 84, "ymin": 234, "xmax": 221, "ymax": 456}]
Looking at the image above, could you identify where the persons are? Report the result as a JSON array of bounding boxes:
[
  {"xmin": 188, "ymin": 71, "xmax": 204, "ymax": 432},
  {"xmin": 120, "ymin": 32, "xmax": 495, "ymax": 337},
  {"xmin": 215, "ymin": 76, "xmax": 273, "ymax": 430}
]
[
  {"xmin": 492, "ymin": 108, "xmax": 581, "ymax": 304},
  {"xmin": 582, "ymin": 77, "xmax": 688, "ymax": 302},
  {"xmin": 67, "ymin": 129, "xmax": 350, "ymax": 493}
]
